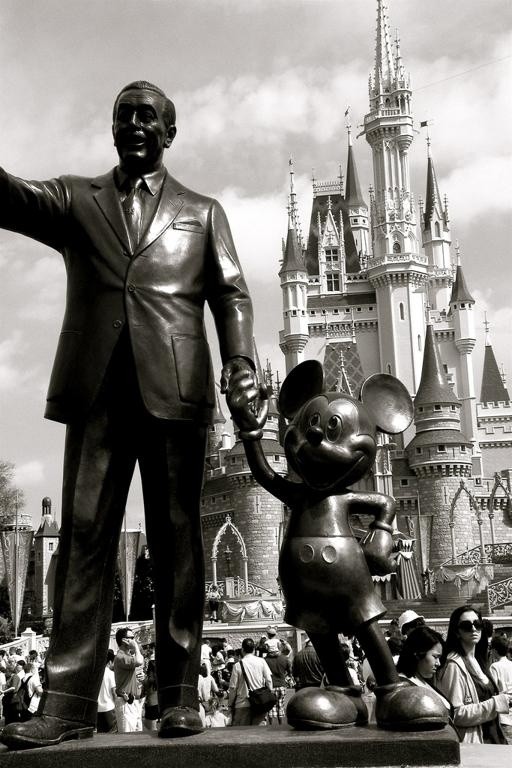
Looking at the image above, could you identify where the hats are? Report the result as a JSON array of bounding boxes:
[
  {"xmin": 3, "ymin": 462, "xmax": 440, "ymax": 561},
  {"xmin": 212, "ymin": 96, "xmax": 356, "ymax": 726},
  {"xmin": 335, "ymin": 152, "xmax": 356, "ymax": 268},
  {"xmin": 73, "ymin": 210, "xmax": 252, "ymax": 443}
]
[{"xmin": 398, "ymin": 609, "xmax": 424, "ymax": 635}]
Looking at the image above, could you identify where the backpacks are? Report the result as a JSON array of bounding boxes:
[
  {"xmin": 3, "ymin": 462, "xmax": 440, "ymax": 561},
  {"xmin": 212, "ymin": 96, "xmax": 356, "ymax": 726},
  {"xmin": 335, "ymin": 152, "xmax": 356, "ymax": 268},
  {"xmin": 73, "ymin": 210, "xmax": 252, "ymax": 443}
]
[{"xmin": 18, "ymin": 676, "xmax": 36, "ymax": 708}]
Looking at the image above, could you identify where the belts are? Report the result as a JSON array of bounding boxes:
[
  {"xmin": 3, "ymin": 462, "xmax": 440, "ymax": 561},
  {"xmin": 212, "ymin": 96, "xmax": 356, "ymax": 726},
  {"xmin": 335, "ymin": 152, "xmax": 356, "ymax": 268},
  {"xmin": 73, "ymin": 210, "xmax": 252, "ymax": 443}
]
[{"xmin": 118, "ymin": 693, "xmax": 142, "ymax": 699}]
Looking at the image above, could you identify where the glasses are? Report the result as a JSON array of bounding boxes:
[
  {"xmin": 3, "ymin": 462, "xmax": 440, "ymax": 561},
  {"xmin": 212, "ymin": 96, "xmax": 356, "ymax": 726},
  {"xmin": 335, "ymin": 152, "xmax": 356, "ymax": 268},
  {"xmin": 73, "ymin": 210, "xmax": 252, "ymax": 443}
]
[{"xmin": 459, "ymin": 620, "xmax": 482, "ymax": 632}]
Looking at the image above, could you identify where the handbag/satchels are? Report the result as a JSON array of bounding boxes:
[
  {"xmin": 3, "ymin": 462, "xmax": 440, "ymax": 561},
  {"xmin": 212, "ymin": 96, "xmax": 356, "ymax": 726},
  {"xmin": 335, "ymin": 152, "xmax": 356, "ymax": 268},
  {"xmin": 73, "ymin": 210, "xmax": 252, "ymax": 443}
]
[{"xmin": 248, "ymin": 687, "xmax": 277, "ymax": 709}]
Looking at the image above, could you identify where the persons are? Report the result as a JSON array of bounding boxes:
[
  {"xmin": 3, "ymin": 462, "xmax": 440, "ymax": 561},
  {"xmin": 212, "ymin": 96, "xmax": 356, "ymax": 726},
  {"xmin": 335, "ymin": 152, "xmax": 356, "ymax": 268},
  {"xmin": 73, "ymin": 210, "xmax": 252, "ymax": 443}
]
[
  {"xmin": 0, "ymin": 647, "xmax": 49, "ymax": 726},
  {"xmin": 0, "ymin": 82, "xmax": 262, "ymax": 748},
  {"xmin": 209, "ymin": 583, "xmax": 218, "ymax": 620},
  {"xmin": 336, "ymin": 605, "xmax": 512, "ymax": 743},
  {"xmin": 97, "ymin": 628, "xmax": 160, "ymax": 733},
  {"xmin": 198, "ymin": 628, "xmax": 330, "ymax": 728}
]
